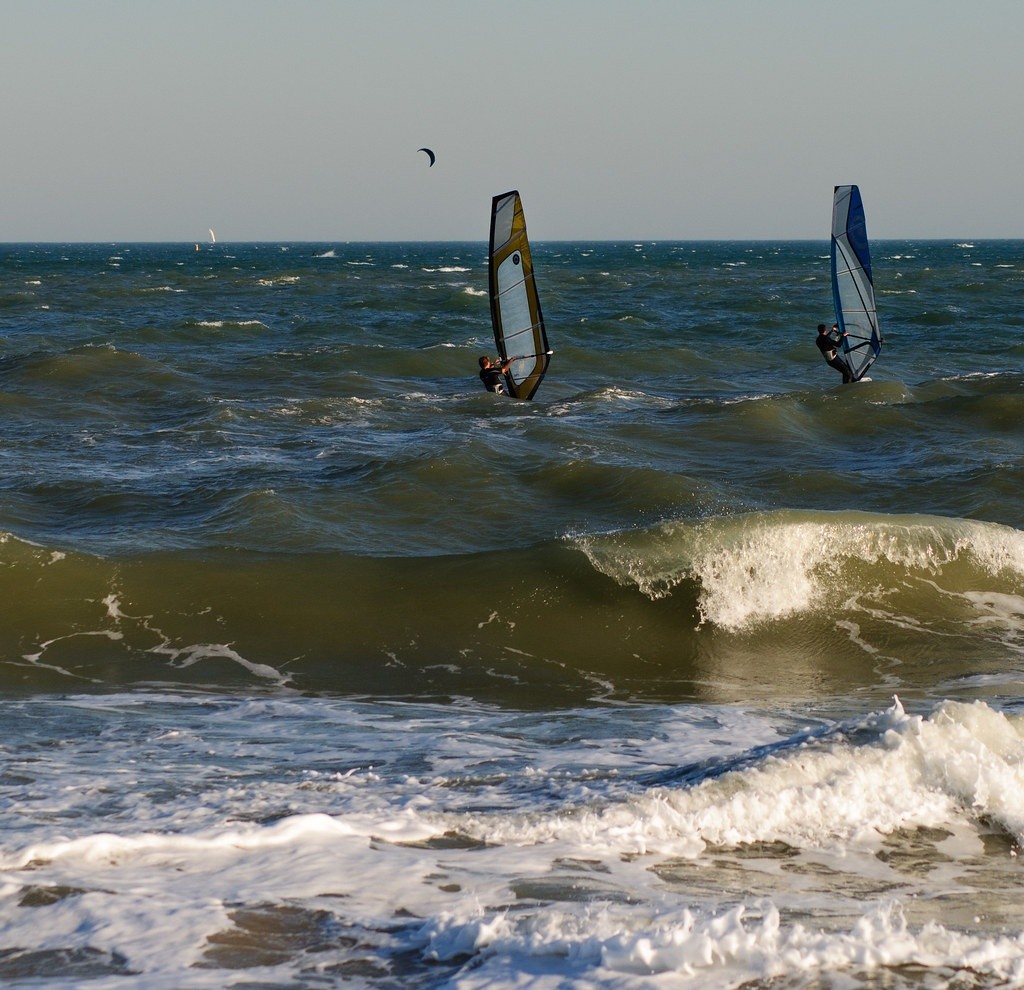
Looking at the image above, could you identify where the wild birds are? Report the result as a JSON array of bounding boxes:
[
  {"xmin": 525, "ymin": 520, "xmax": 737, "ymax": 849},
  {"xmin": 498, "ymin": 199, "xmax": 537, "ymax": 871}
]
[{"xmin": 417, "ymin": 148, "xmax": 436, "ymax": 167}]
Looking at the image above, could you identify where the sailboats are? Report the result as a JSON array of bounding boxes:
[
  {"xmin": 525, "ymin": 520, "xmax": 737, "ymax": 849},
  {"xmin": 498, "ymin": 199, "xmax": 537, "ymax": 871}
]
[
  {"xmin": 485, "ymin": 191, "xmax": 553, "ymax": 398},
  {"xmin": 830, "ymin": 183, "xmax": 882, "ymax": 385}
]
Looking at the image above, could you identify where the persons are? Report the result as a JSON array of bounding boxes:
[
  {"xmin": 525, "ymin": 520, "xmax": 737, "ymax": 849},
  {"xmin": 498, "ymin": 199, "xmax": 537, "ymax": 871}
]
[
  {"xmin": 814, "ymin": 323, "xmax": 852, "ymax": 385},
  {"xmin": 478, "ymin": 354, "xmax": 517, "ymax": 397}
]
